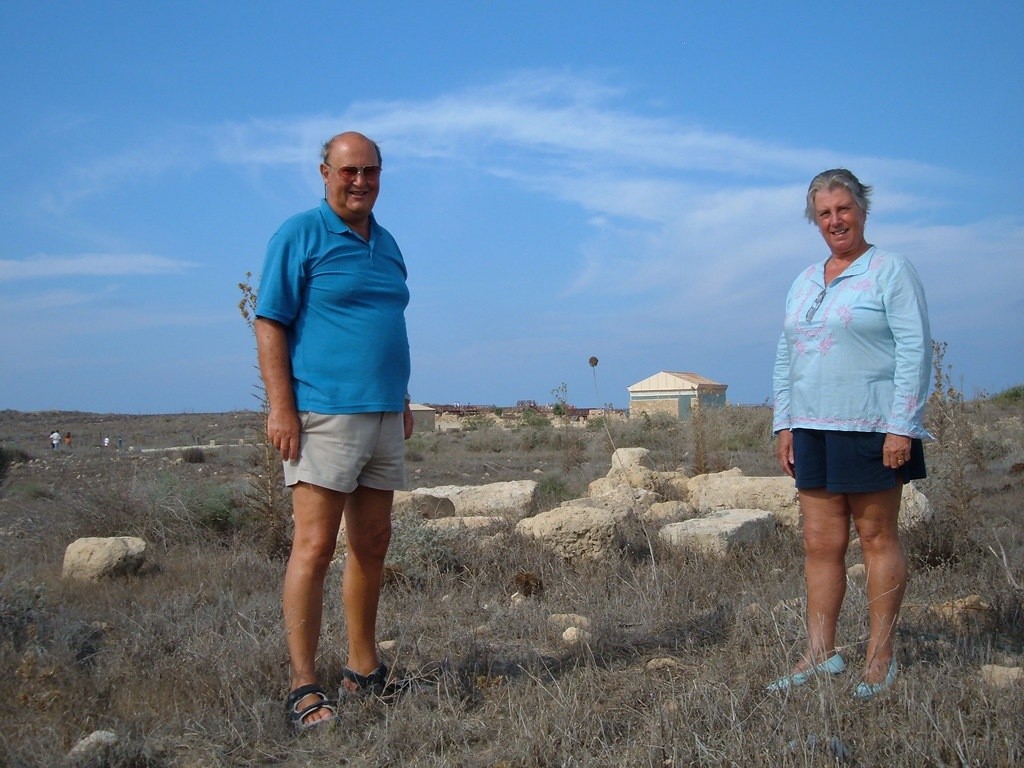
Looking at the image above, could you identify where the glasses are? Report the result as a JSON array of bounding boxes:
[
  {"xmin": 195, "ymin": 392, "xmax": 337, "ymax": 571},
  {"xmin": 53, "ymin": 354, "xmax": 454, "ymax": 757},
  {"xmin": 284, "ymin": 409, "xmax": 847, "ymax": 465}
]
[
  {"xmin": 805, "ymin": 289, "xmax": 826, "ymax": 322},
  {"xmin": 329, "ymin": 166, "xmax": 382, "ymax": 180}
]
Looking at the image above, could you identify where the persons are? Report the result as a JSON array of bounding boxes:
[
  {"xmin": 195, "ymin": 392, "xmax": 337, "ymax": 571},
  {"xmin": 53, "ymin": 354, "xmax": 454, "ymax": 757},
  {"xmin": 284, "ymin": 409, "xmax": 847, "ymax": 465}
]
[
  {"xmin": 765, "ymin": 168, "xmax": 932, "ymax": 702},
  {"xmin": 49, "ymin": 429, "xmax": 122, "ymax": 453},
  {"xmin": 255, "ymin": 132, "xmax": 415, "ymax": 731}
]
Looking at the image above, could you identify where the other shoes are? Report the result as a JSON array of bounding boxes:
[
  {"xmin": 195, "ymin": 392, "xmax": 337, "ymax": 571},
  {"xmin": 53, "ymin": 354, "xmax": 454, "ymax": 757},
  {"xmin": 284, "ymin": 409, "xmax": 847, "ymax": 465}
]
[
  {"xmin": 849, "ymin": 656, "xmax": 898, "ymax": 702},
  {"xmin": 762, "ymin": 653, "xmax": 844, "ymax": 697}
]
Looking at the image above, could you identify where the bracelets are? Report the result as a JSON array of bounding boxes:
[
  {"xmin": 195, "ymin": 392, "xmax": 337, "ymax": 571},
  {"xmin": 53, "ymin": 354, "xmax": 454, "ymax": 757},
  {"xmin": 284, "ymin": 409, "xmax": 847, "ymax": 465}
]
[{"xmin": 405, "ymin": 392, "xmax": 411, "ymax": 403}]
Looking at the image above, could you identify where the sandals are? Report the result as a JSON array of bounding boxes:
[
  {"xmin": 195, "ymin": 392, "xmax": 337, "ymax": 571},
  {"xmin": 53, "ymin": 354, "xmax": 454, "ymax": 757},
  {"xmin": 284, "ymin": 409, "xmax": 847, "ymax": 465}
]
[
  {"xmin": 338, "ymin": 664, "xmax": 426, "ymax": 706},
  {"xmin": 287, "ymin": 683, "xmax": 340, "ymax": 731}
]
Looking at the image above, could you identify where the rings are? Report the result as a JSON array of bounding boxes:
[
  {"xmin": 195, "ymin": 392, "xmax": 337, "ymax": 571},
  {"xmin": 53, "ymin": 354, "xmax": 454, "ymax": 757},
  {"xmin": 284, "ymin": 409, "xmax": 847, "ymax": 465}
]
[{"xmin": 897, "ymin": 459, "xmax": 903, "ymax": 462}]
